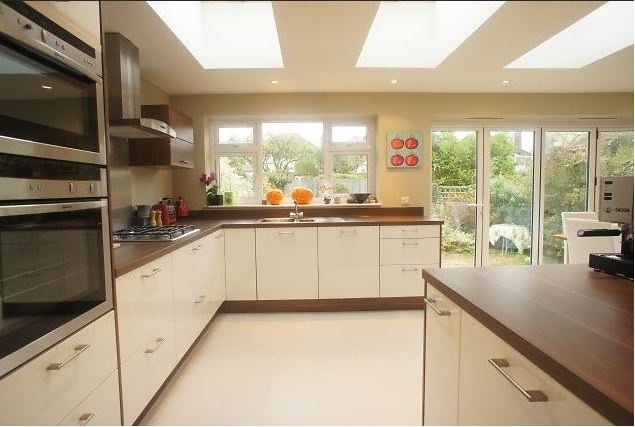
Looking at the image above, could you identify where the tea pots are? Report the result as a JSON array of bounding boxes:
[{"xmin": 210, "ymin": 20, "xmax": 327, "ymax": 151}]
[{"xmin": 175, "ymin": 196, "xmax": 190, "ymax": 221}]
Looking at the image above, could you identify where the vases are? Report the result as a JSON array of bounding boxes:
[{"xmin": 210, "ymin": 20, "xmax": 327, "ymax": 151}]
[{"xmin": 206, "ymin": 193, "xmax": 224, "ymax": 206}]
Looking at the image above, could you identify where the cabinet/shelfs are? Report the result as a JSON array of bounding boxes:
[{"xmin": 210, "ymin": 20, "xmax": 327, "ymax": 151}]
[
  {"xmin": 129, "ymin": 104, "xmax": 195, "ymax": 169},
  {"xmin": 379, "ymin": 224, "xmax": 444, "ymax": 311},
  {"xmin": 318, "ymin": 225, "xmax": 380, "ymax": 311},
  {"xmin": 421, "ymin": 263, "xmax": 631, "ymax": 426},
  {"xmin": 256, "ymin": 226, "xmax": 319, "ymax": 310},
  {"xmin": 172, "ymin": 228, "xmax": 257, "ymax": 379},
  {"xmin": 113, "ymin": 253, "xmax": 178, "ymax": 425}
]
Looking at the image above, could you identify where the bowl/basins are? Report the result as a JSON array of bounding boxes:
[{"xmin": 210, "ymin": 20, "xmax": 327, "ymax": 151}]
[{"xmin": 350, "ymin": 193, "xmax": 369, "ymax": 203}]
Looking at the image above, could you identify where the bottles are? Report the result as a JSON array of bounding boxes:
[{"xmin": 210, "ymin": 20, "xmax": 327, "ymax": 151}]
[
  {"xmin": 151, "ymin": 211, "xmax": 156, "ymax": 226},
  {"xmin": 157, "ymin": 211, "xmax": 162, "ymax": 226}
]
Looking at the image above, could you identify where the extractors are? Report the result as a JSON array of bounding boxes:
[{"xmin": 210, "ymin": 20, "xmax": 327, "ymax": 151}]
[{"xmin": 105, "ymin": 32, "xmax": 176, "ymax": 139}]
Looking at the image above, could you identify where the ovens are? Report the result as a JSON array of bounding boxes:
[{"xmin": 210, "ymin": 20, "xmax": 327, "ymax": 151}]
[{"xmin": 0, "ymin": 2, "xmax": 115, "ymax": 377}]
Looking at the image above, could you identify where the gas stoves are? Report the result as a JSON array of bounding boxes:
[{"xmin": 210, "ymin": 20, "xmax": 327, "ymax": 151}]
[{"xmin": 113, "ymin": 225, "xmax": 201, "ymax": 242}]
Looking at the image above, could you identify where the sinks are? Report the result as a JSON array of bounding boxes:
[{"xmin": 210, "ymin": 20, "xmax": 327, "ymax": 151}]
[{"xmin": 258, "ymin": 217, "xmax": 315, "ymax": 222}]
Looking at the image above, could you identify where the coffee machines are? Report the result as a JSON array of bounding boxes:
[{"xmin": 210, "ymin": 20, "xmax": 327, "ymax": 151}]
[{"xmin": 576, "ymin": 176, "xmax": 634, "ymax": 277}]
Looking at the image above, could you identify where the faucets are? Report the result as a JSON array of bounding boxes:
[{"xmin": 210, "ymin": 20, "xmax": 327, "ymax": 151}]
[{"xmin": 294, "ymin": 200, "xmax": 299, "ymax": 220}]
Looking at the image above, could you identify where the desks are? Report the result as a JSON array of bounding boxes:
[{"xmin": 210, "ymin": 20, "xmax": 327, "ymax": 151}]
[{"xmin": 489, "ymin": 222, "xmax": 531, "ymax": 259}]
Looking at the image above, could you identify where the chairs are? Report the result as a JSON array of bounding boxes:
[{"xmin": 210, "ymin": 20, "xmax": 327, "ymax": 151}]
[
  {"xmin": 564, "ymin": 217, "xmax": 625, "ymax": 265},
  {"xmin": 562, "ymin": 210, "xmax": 600, "ymax": 264}
]
